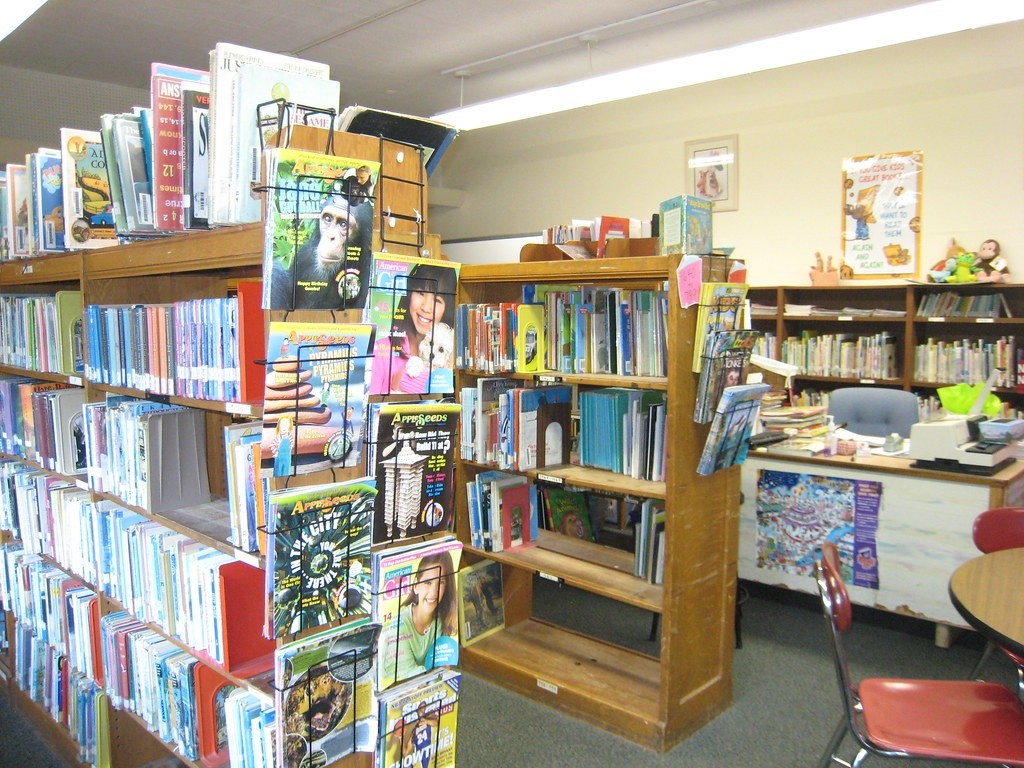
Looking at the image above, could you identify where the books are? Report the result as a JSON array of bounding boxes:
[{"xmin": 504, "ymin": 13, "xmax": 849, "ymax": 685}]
[
  {"xmin": 458, "ymin": 196, "xmax": 771, "ymax": 651},
  {"xmin": 747, "ymin": 292, "xmax": 1024, "ymax": 425},
  {"xmin": 0, "ymin": 43, "xmax": 460, "ymax": 768}
]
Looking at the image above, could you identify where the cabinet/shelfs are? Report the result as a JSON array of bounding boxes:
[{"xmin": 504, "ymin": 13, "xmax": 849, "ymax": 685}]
[
  {"xmin": 745, "ymin": 282, "xmax": 1024, "ymax": 407},
  {"xmin": 441, "ymin": 238, "xmax": 745, "ymax": 754},
  {"xmin": 256, "ymin": 98, "xmax": 375, "ymax": 768},
  {"xmin": 695, "ymin": 252, "xmax": 759, "ymax": 474},
  {"xmin": 0, "ymin": 127, "xmax": 440, "ymax": 768},
  {"xmin": 363, "ymin": 133, "xmax": 458, "ymax": 768}
]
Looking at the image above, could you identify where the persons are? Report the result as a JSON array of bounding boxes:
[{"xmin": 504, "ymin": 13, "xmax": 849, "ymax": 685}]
[
  {"xmin": 394, "ymin": 700, "xmax": 438, "ymax": 768},
  {"xmin": 370, "ymin": 264, "xmax": 458, "ymax": 395},
  {"xmin": 377, "ymin": 550, "xmax": 457, "ymax": 680}
]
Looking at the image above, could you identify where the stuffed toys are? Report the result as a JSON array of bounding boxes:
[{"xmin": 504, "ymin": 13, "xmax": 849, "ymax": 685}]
[{"xmin": 926, "ymin": 237, "xmax": 1011, "ymax": 284}]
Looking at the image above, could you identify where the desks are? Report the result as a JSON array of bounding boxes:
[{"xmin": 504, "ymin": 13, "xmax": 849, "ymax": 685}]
[
  {"xmin": 948, "ymin": 547, "xmax": 1024, "ymax": 659},
  {"xmin": 737, "ymin": 445, "xmax": 1024, "ymax": 648}
]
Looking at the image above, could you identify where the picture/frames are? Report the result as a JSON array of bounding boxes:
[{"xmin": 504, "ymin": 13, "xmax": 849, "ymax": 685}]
[{"xmin": 684, "ymin": 134, "xmax": 739, "ymax": 212}]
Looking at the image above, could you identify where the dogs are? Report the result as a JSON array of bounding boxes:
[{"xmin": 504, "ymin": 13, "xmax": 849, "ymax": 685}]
[{"xmin": 405, "ymin": 322, "xmax": 454, "ymax": 379}]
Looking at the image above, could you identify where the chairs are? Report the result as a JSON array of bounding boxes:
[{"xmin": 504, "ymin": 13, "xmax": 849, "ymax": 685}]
[
  {"xmin": 966, "ymin": 506, "xmax": 1024, "ymax": 702},
  {"xmin": 827, "ymin": 387, "xmax": 920, "ymax": 437},
  {"xmin": 814, "ymin": 541, "xmax": 1024, "ymax": 768}
]
